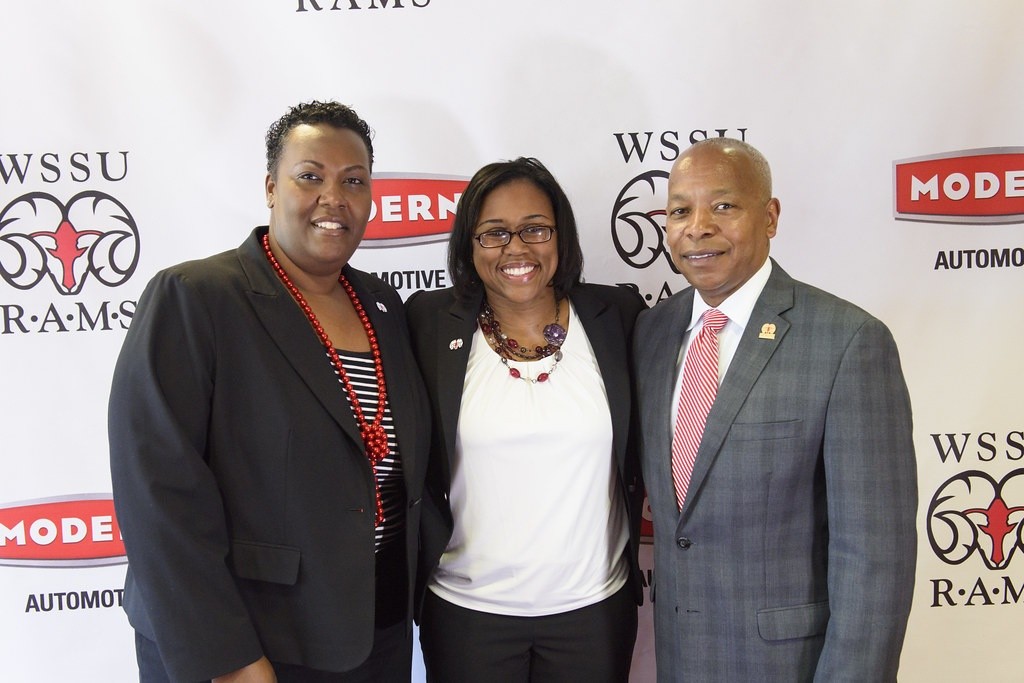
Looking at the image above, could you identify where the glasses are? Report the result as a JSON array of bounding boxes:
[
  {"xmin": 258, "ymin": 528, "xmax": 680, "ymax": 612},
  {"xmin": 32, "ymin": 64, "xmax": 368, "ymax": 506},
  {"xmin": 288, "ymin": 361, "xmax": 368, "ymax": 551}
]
[{"xmin": 474, "ymin": 226, "xmax": 556, "ymax": 248}]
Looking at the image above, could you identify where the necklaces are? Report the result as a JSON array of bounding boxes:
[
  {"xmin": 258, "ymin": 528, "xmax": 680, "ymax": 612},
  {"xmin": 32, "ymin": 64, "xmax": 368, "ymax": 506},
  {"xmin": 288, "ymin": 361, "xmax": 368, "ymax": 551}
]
[
  {"xmin": 263, "ymin": 234, "xmax": 390, "ymax": 528},
  {"xmin": 478, "ymin": 291, "xmax": 567, "ymax": 382}
]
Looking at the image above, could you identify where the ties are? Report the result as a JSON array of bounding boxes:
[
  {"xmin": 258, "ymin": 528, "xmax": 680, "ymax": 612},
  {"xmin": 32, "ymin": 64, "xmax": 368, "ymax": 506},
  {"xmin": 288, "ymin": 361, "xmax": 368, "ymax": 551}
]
[{"xmin": 671, "ymin": 311, "xmax": 729, "ymax": 513}]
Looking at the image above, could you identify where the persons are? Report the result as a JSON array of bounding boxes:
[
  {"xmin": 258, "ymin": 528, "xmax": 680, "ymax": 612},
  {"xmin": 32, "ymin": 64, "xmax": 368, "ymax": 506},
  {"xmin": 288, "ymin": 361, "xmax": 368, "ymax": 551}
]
[
  {"xmin": 404, "ymin": 157, "xmax": 651, "ymax": 683},
  {"xmin": 632, "ymin": 138, "xmax": 918, "ymax": 683},
  {"xmin": 108, "ymin": 100, "xmax": 432, "ymax": 683}
]
